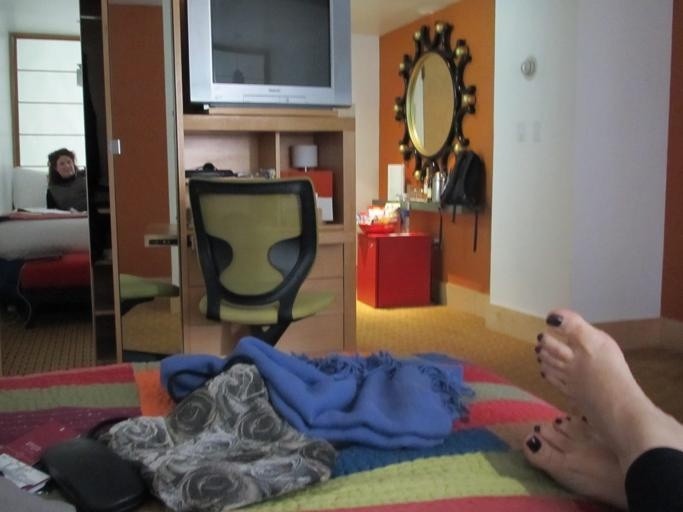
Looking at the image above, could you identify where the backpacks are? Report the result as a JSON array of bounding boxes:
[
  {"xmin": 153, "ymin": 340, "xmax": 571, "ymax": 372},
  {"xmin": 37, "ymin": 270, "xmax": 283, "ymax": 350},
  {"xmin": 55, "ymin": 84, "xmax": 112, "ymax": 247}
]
[{"xmin": 440, "ymin": 149, "xmax": 487, "ymax": 210}]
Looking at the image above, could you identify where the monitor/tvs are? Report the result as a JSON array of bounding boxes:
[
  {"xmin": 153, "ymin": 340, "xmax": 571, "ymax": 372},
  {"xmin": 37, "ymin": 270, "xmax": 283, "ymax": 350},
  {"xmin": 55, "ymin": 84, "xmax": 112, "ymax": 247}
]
[{"xmin": 185, "ymin": 0, "xmax": 353, "ymax": 113}]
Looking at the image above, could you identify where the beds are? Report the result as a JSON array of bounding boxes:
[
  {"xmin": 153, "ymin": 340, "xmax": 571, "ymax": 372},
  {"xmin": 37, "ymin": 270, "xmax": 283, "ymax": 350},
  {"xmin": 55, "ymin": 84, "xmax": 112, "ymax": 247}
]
[
  {"xmin": 2, "ymin": 165, "xmax": 92, "ymax": 261},
  {"xmin": 1, "ymin": 352, "xmax": 622, "ymax": 510}
]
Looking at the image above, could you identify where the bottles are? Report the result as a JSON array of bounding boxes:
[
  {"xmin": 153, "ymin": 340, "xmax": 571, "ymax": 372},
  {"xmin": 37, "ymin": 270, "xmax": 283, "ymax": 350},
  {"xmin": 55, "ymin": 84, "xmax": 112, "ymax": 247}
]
[{"xmin": 400, "ymin": 194, "xmax": 409, "ymax": 234}]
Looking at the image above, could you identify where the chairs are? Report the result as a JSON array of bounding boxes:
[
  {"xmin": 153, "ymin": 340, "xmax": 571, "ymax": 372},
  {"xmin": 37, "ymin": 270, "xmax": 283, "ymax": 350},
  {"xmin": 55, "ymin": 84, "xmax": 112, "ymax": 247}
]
[{"xmin": 187, "ymin": 175, "xmax": 336, "ymax": 348}]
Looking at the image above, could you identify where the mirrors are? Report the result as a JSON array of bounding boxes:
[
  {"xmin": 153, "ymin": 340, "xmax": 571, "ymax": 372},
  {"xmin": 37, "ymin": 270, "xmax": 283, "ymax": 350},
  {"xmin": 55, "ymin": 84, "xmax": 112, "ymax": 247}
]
[
  {"xmin": 1, "ymin": 1, "xmax": 128, "ymax": 380},
  {"xmin": 393, "ymin": 20, "xmax": 478, "ymax": 186}
]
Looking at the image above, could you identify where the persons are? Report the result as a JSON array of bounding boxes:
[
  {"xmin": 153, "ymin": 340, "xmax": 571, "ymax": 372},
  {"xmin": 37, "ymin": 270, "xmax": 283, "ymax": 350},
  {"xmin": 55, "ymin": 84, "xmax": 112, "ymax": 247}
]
[
  {"xmin": 516, "ymin": 305, "xmax": 683, "ymax": 511},
  {"xmin": 43, "ymin": 147, "xmax": 86, "ymax": 213}
]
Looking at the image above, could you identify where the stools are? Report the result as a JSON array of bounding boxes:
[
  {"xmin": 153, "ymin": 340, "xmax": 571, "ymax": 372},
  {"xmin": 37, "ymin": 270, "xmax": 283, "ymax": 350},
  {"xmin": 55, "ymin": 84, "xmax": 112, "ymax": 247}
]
[{"xmin": 15, "ymin": 253, "xmax": 92, "ymax": 331}]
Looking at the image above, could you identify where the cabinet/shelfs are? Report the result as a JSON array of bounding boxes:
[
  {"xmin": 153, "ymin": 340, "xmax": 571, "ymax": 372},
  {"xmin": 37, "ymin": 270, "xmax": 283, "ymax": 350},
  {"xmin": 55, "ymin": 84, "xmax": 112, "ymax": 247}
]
[
  {"xmin": 182, "ymin": 112, "xmax": 358, "ymax": 358},
  {"xmin": 357, "ymin": 229, "xmax": 433, "ymax": 308}
]
